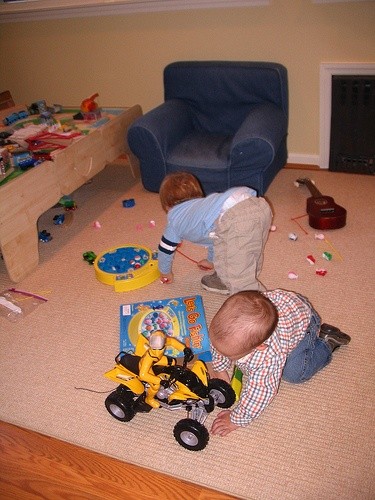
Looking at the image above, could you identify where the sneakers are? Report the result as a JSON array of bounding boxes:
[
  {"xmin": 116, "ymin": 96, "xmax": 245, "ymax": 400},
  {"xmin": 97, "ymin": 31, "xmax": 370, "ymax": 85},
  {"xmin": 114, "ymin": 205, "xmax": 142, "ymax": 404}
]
[
  {"xmin": 201, "ymin": 272, "xmax": 230, "ymax": 294},
  {"xmin": 319, "ymin": 323, "xmax": 351, "ymax": 352}
]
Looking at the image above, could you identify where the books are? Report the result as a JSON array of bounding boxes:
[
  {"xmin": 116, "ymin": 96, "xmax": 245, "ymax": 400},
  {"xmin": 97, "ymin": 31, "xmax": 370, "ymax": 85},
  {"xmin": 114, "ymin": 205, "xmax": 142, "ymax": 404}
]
[{"xmin": 119, "ymin": 295, "xmax": 211, "ymax": 362}]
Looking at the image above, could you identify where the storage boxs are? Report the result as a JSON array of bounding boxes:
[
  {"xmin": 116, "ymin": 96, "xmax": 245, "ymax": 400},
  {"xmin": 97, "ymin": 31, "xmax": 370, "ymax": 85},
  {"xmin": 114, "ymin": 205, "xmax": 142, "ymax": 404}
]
[{"xmin": 120, "ymin": 296, "xmax": 212, "ymax": 363}]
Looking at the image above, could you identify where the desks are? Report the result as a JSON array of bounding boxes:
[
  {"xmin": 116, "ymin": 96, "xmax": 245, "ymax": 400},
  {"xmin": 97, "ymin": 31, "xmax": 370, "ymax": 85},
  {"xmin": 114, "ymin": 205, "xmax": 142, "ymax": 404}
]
[{"xmin": 0, "ymin": 104, "xmax": 143, "ymax": 284}]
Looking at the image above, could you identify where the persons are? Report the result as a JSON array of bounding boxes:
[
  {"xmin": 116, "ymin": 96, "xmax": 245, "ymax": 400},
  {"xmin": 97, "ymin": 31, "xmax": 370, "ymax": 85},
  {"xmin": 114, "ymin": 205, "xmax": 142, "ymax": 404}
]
[
  {"xmin": 158, "ymin": 171, "xmax": 273, "ymax": 296},
  {"xmin": 138, "ymin": 332, "xmax": 194, "ymax": 409},
  {"xmin": 208, "ymin": 288, "xmax": 352, "ymax": 437}
]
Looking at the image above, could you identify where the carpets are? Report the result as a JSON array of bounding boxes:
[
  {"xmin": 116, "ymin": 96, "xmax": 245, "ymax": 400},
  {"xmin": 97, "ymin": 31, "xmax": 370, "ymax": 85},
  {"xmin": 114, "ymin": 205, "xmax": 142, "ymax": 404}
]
[{"xmin": 0, "ymin": 150, "xmax": 375, "ymax": 500}]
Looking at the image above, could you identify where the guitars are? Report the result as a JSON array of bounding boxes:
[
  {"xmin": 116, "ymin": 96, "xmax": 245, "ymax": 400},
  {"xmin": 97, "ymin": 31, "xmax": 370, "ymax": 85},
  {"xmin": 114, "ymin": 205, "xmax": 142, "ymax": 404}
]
[{"xmin": 295, "ymin": 177, "xmax": 347, "ymax": 230}]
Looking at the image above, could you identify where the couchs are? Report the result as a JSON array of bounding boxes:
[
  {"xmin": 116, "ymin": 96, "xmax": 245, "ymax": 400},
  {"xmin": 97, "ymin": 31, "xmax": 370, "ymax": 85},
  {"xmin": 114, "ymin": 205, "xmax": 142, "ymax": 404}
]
[{"xmin": 127, "ymin": 61, "xmax": 289, "ymax": 198}]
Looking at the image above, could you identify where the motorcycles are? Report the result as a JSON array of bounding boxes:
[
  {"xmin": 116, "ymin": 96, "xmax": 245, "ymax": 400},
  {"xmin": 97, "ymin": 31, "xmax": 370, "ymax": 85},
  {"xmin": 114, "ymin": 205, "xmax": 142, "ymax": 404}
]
[{"xmin": 105, "ymin": 340, "xmax": 238, "ymax": 451}]
